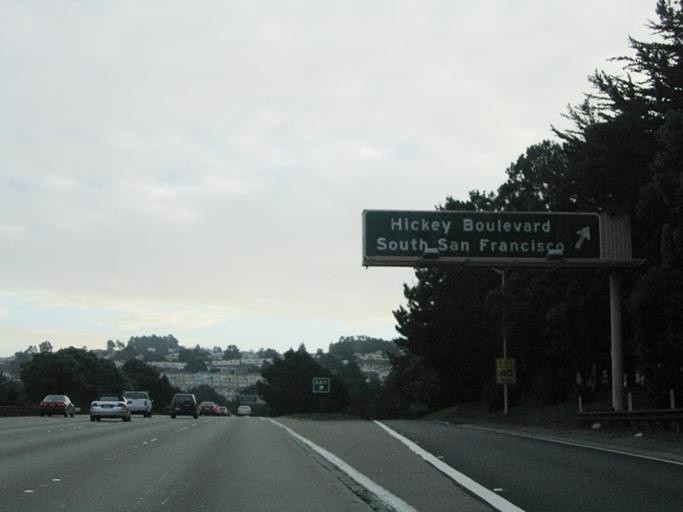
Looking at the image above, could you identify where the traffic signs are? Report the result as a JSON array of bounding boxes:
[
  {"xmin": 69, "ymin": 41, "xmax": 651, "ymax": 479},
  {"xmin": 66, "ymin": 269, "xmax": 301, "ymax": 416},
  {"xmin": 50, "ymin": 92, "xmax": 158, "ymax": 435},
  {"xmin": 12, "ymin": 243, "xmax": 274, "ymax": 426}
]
[
  {"xmin": 360, "ymin": 206, "xmax": 605, "ymax": 261},
  {"xmin": 495, "ymin": 367, "xmax": 517, "ymax": 385},
  {"xmin": 312, "ymin": 377, "xmax": 331, "ymax": 394}
]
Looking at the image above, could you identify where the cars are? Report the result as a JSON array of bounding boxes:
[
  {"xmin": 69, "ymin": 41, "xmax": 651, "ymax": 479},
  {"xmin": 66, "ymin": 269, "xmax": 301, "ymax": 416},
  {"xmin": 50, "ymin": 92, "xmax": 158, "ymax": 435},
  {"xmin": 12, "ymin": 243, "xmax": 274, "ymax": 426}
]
[{"xmin": 36, "ymin": 390, "xmax": 252, "ymax": 422}]
